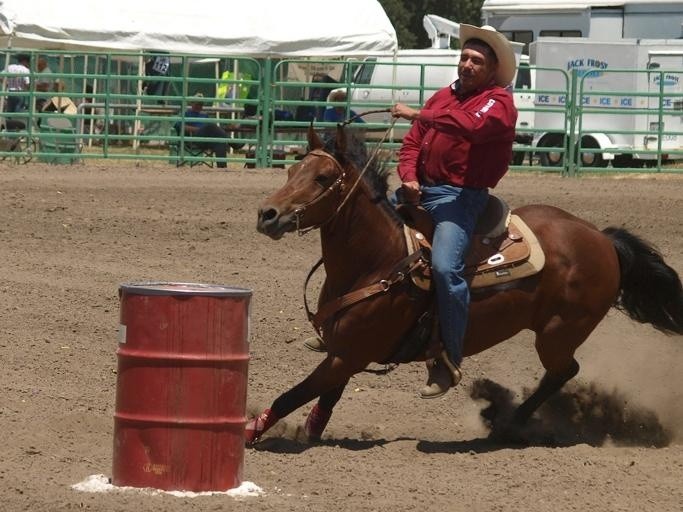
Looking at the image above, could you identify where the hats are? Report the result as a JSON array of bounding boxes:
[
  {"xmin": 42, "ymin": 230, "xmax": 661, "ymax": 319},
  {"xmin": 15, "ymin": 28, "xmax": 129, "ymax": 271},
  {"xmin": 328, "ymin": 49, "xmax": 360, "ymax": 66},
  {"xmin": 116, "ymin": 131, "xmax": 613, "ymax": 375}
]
[
  {"xmin": 459, "ymin": 23, "xmax": 516, "ymax": 88},
  {"xmin": 326, "ymin": 87, "xmax": 347, "ymax": 110}
]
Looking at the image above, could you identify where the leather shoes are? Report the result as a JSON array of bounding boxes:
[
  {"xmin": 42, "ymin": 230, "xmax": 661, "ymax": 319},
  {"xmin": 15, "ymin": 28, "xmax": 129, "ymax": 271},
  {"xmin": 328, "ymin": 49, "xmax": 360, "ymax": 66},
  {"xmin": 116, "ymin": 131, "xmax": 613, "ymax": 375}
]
[
  {"xmin": 303, "ymin": 334, "xmax": 329, "ymax": 352},
  {"xmin": 419, "ymin": 359, "xmax": 462, "ymax": 399}
]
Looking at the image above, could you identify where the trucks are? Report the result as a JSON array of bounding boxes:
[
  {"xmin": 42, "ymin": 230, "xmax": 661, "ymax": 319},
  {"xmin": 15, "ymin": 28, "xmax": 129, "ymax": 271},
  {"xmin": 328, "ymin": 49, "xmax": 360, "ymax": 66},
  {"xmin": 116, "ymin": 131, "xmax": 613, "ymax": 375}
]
[{"xmin": 320, "ymin": 44, "xmax": 536, "ymax": 166}]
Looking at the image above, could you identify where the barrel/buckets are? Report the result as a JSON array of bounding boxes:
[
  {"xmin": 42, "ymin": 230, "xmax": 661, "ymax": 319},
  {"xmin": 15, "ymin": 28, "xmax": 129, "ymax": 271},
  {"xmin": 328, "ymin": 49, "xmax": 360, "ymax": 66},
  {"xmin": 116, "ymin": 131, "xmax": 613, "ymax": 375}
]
[{"xmin": 113, "ymin": 281, "xmax": 254, "ymax": 489}]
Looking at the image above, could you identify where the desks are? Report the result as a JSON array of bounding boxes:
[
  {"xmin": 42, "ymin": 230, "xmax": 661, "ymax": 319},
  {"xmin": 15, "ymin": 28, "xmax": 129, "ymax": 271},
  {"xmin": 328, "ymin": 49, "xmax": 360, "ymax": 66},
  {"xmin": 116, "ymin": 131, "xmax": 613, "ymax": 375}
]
[
  {"xmin": 224, "ymin": 124, "xmax": 386, "ymax": 154},
  {"xmin": 135, "ymin": 108, "xmax": 230, "ymax": 142}
]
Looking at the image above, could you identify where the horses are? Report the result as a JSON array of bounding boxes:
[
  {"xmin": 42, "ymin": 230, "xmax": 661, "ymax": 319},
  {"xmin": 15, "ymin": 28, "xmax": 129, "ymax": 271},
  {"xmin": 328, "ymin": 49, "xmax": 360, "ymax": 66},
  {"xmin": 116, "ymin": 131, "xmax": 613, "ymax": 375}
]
[{"xmin": 242, "ymin": 116, "xmax": 683, "ymax": 451}]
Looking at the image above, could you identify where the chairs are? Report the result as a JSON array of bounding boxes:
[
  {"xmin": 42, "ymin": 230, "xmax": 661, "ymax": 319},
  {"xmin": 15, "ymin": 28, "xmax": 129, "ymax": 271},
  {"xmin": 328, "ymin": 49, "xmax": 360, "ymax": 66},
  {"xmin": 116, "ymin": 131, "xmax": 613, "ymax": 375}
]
[
  {"xmin": 0, "ymin": 119, "xmax": 35, "ymax": 165},
  {"xmin": 176, "ymin": 132, "xmax": 213, "ymax": 168}
]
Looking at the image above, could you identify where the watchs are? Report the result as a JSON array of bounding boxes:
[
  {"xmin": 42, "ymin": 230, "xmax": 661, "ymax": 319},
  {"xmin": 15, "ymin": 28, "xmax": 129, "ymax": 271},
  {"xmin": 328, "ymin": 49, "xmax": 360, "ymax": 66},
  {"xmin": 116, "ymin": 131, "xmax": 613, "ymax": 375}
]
[{"xmin": 0, "ymin": 52, "xmax": 31, "ymax": 151}]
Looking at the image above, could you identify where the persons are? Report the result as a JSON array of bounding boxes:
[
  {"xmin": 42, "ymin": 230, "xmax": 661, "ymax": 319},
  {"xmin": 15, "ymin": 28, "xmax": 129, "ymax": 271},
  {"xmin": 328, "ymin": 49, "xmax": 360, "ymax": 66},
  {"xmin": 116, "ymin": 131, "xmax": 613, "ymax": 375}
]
[
  {"xmin": 36, "ymin": 57, "xmax": 53, "ymax": 111},
  {"xmin": 175, "ymin": 93, "xmax": 245, "ymax": 169},
  {"xmin": 140, "ymin": 49, "xmax": 171, "ymax": 106},
  {"xmin": 75, "ymin": 84, "xmax": 104, "ymax": 147},
  {"xmin": 40, "ymin": 79, "xmax": 78, "ymax": 116},
  {"xmin": 321, "ymin": 89, "xmax": 367, "ymax": 167},
  {"xmin": 301, "ymin": 20, "xmax": 519, "ymax": 398}
]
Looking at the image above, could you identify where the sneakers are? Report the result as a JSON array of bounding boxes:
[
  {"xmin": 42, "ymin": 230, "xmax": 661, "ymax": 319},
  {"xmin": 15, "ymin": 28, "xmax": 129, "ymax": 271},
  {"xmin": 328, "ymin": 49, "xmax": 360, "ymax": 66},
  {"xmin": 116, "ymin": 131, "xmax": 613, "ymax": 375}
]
[{"xmin": 232, "ymin": 143, "xmax": 245, "ymax": 149}]
